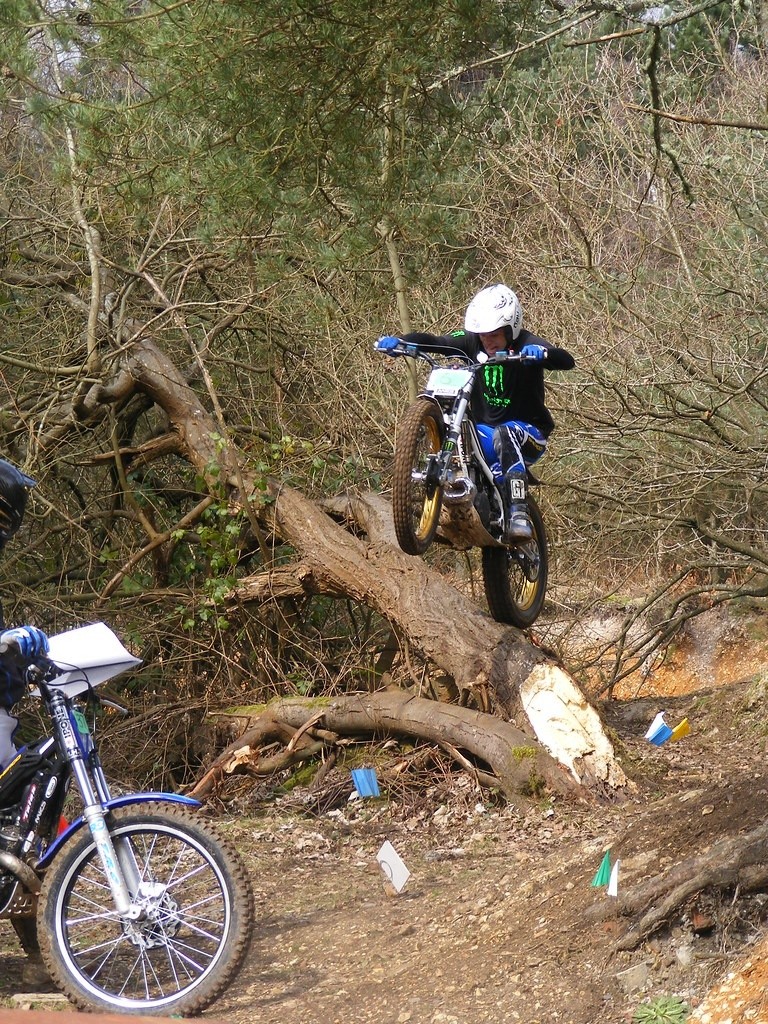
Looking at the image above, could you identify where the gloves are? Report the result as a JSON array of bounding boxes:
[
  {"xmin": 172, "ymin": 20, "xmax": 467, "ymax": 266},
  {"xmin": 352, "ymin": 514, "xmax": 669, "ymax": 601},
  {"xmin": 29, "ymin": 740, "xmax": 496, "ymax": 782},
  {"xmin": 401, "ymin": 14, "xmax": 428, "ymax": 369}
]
[
  {"xmin": 0, "ymin": 626, "xmax": 50, "ymax": 657},
  {"xmin": 373, "ymin": 336, "xmax": 404, "ymax": 356},
  {"xmin": 521, "ymin": 344, "xmax": 548, "ymax": 365}
]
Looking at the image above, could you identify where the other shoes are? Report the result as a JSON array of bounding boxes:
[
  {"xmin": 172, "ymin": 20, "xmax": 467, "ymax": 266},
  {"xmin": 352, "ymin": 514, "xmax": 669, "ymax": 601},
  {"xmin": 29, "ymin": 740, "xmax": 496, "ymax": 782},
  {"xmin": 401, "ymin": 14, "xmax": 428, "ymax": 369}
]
[{"xmin": 23, "ymin": 956, "xmax": 101, "ymax": 992}]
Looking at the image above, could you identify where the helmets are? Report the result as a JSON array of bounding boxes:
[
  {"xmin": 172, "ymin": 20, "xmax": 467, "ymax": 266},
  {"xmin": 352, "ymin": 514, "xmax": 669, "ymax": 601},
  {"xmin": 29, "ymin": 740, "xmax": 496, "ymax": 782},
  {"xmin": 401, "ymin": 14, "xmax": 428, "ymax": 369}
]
[
  {"xmin": 464, "ymin": 283, "xmax": 522, "ymax": 340},
  {"xmin": 0, "ymin": 458, "xmax": 39, "ymax": 549}
]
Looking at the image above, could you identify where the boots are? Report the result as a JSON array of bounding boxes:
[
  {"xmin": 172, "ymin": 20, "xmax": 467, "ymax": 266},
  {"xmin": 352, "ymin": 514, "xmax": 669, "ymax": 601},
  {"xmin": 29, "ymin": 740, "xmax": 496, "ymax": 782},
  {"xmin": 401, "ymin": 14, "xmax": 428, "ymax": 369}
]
[{"xmin": 505, "ymin": 471, "xmax": 532, "ymax": 543}]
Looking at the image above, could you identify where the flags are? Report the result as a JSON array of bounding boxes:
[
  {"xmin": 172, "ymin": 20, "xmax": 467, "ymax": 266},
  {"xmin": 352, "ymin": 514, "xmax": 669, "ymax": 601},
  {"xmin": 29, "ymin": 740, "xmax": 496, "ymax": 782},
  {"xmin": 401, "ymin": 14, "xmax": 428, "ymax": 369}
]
[
  {"xmin": 646, "ymin": 712, "xmax": 689, "ymax": 746},
  {"xmin": 58, "ymin": 816, "xmax": 69, "ymax": 834},
  {"xmin": 589, "ymin": 849, "xmax": 620, "ymax": 896},
  {"xmin": 351, "ymin": 769, "xmax": 380, "ymax": 798},
  {"xmin": 376, "ymin": 840, "xmax": 410, "ymax": 891}
]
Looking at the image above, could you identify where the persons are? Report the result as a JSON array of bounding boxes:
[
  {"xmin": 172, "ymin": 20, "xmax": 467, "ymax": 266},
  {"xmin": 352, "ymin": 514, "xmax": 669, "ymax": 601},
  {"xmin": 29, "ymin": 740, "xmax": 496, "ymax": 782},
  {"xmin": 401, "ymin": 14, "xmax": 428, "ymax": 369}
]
[
  {"xmin": 377, "ymin": 284, "xmax": 574, "ymax": 539},
  {"xmin": 0, "ymin": 459, "xmax": 50, "ymax": 964}
]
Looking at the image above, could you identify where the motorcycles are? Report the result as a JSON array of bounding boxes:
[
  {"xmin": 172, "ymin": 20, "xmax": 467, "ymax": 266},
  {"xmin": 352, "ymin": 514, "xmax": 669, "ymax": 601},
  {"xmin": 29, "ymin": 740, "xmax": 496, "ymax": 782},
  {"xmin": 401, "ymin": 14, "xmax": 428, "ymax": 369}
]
[
  {"xmin": 374, "ymin": 337, "xmax": 548, "ymax": 629},
  {"xmin": 0, "ymin": 642, "xmax": 254, "ymax": 1018}
]
